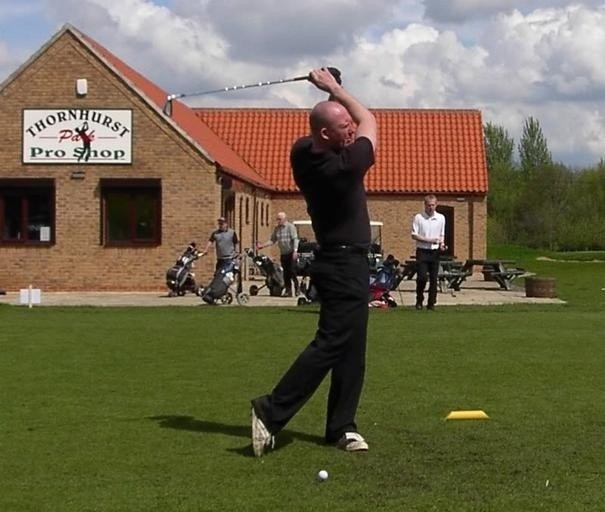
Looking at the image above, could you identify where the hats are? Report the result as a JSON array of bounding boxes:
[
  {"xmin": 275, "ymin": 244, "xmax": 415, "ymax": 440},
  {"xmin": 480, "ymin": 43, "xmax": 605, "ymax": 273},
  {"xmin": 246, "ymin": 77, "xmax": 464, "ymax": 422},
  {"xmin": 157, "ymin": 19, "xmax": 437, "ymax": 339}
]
[{"xmin": 218, "ymin": 217, "xmax": 227, "ymax": 222}]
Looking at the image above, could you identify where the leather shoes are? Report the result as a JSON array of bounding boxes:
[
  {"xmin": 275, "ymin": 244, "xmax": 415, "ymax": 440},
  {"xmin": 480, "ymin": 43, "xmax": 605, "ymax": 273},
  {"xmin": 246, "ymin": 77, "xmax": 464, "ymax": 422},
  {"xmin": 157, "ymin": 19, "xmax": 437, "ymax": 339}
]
[
  {"xmin": 416, "ymin": 304, "xmax": 435, "ymax": 311},
  {"xmin": 283, "ymin": 288, "xmax": 300, "ymax": 297}
]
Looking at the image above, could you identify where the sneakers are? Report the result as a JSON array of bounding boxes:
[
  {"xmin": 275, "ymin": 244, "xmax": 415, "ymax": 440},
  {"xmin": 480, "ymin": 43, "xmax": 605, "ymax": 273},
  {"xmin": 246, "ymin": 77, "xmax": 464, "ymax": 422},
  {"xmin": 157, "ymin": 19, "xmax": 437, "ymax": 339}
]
[
  {"xmin": 338, "ymin": 432, "xmax": 369, "ymax": 450},
  {"xmin": 251, "ymin": 407, "xmax": 274, "ymax": 456}
]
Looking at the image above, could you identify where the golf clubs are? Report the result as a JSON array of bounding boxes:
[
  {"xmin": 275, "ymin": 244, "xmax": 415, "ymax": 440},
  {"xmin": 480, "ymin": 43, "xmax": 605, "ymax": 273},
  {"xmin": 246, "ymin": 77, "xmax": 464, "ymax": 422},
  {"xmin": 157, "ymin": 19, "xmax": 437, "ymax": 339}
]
[{"xmin": 162, "ymin": 68, "xmax": 342, "ymax": 117}]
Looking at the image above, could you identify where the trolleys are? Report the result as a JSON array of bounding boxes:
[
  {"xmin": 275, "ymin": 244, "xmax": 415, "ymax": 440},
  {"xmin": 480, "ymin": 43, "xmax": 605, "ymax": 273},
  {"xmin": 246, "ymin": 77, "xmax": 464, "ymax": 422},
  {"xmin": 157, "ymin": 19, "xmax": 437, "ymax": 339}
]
[
  {"xmin": 369, "ymin": 256, "xmax": 403, "ymax": 307},
  {"xmin": 164, "ymin": 243, "xmax": 314, "ymax": 306}
]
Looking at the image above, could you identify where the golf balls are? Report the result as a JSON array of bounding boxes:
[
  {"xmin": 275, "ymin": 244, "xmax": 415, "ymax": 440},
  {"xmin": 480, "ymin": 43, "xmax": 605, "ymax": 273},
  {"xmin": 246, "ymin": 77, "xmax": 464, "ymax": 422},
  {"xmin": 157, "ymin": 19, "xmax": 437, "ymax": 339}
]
[{"xmin": 318, "ymin": 470, "xmax": 328, "ymax": 480}]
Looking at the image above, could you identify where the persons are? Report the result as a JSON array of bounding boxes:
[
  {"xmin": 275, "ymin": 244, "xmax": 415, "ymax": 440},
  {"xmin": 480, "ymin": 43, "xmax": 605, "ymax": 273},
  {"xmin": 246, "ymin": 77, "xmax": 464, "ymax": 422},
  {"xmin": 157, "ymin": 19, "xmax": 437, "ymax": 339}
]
[
  {"xmin": 251, "ymin": 66, "xmax": 377, "ymax": 457},
  {"xmin": 256, "ymin": 212, "xmax": 300, "ymax": 296},
  {"xmin": 203, "ymin": 217, "xmax": 239, "ymax": 272},
  {"xmin": 75, "ymin": 121, "xmax": 96, "ymax": 162},
  {"xmin": 411, "ymin": 195, "xmax": 448, "ymax": 311},
  {"xmin": 383, "ymin": 255, "xmax": 399, "ymax": 268}
]
